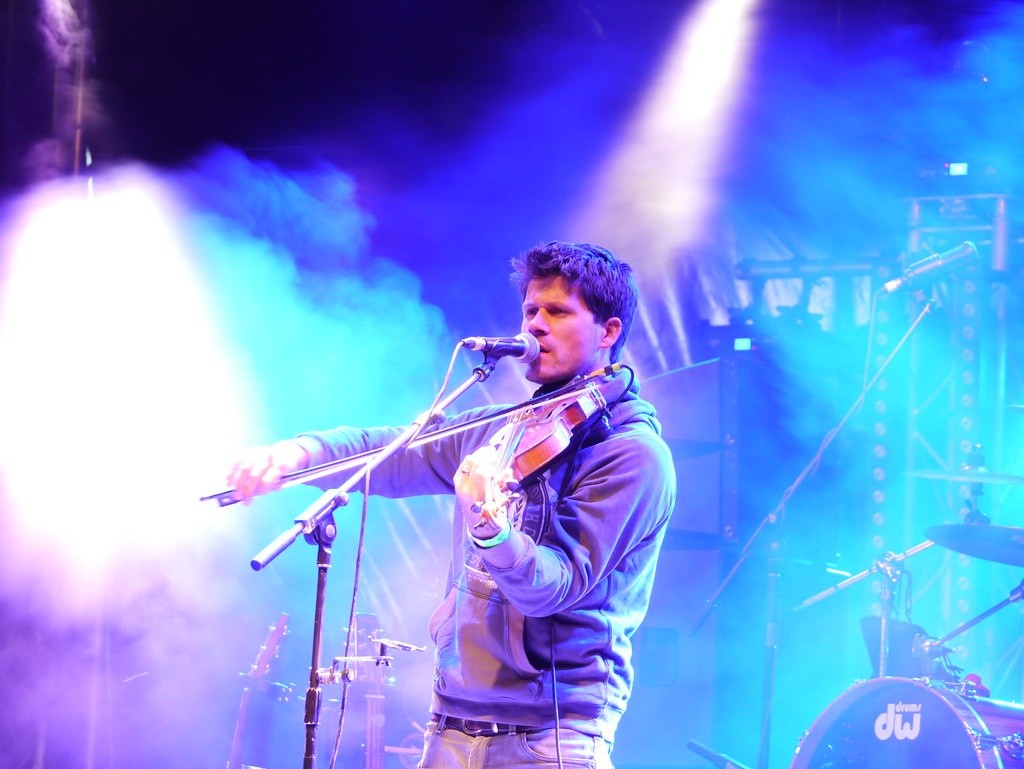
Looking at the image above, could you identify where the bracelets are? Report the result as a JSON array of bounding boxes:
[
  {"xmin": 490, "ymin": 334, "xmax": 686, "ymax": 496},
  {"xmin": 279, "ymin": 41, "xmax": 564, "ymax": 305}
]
[{"xmin": 468, "ymin": 522, "xmax": 510, "ymax": 547}]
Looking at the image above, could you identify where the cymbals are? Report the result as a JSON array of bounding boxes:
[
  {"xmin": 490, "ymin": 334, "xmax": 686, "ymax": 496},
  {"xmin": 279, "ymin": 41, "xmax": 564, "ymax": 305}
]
[
  {"xmin": 1004, "ymin": 403, "xmax": 1024, "ymax": 417},
  {"xmin": 924, "ymin": 524, "xmax": 1024, "ymax": 567},
  {"xmin": 901, "ymin": 468, "xmax": 1024, "ymax": 483}
]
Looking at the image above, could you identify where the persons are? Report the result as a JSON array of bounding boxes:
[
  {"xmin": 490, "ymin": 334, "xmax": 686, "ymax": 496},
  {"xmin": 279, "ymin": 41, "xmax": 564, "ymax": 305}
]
[{"xmin": 226, "ymin": 240, "xmax": 678, "ymax": 769}]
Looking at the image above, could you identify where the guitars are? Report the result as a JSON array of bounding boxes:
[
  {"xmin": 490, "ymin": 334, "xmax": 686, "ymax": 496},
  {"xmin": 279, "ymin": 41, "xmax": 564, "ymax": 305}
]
[
  {"xmin": 227, "ymin": 610, "xmax": 289, "ymax": 769},
  {"xmin": 349, "ymin": 612, "xmax": 391, "ymax": 769}
]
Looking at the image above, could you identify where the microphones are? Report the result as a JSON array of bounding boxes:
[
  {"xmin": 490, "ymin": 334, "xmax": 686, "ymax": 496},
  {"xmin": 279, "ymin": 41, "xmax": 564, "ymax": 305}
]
[
  {"xmin": 462, "ymin": 332, "xmax": 540, "ymax": 363},
  {"xmin": 991, "ymin": 200, "xmax": 1005, "ymax": 270},
  {"xmin": 882, "ymin": 241, "xmax": 979, "ymax": 293}
]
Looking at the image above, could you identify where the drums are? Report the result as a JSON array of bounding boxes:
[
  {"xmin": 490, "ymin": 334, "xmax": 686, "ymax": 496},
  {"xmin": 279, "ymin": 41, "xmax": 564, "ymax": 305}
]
[{"xmin": 785, "ymin": 673, "xmax": 1024, "ymax": 769}]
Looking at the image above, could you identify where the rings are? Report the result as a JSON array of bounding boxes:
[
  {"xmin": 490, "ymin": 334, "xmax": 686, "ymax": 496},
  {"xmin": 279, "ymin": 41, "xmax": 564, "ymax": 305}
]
[{"xmin": 462, "ymin": 469, "xmax": 471, "ymax": 477}]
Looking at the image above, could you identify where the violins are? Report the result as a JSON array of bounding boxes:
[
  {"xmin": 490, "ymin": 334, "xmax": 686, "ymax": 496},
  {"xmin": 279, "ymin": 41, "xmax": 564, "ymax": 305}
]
[{"xmin": 475, "ymin": 377, "xmax": 625, "ymax": 527}]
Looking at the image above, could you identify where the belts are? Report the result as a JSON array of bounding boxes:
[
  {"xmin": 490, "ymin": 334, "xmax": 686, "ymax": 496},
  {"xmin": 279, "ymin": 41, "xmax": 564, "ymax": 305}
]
[{"xmin": 431, "ymin": 713, "xmax": 538, "ymax": 737}]
